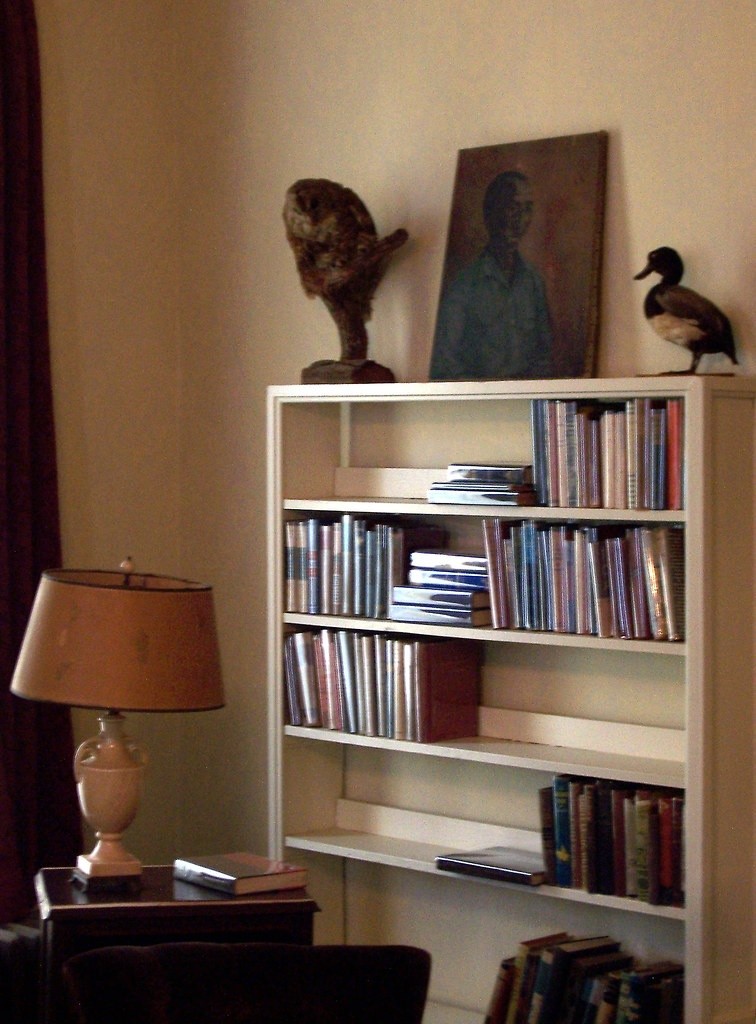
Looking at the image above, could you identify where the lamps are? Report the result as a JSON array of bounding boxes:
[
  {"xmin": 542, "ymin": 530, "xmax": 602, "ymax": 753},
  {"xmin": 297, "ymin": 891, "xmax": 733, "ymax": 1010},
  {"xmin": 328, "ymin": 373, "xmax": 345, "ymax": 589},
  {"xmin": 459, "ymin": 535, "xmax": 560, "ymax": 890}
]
[{"xmin": 11, "ymin": 557, "xmax": 233, "ymax": 896}]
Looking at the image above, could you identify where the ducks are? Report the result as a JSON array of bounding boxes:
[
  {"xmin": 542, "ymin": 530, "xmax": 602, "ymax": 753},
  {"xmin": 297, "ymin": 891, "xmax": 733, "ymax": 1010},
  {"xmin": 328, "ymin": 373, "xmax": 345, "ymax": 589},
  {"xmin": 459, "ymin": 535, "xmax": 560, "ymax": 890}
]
[{"xmin": 635, "ymin": 245, "xmax": 740, "ymax": 376}]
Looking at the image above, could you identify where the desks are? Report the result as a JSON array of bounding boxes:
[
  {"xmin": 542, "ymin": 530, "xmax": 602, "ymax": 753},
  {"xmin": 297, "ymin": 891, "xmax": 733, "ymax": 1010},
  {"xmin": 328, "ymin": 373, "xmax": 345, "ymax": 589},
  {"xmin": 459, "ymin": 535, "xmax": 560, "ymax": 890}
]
[{"xmin": 34, "ymin": 866, "xmax": 322, "ymax": 1024}]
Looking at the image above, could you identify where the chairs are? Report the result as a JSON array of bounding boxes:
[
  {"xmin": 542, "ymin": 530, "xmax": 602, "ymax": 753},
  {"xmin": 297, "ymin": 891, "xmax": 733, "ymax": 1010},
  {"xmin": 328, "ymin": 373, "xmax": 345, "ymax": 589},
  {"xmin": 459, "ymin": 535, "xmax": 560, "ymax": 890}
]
[{"xmin": 61, "ymin": 942, "xmax": 431, "ymax": 1024}]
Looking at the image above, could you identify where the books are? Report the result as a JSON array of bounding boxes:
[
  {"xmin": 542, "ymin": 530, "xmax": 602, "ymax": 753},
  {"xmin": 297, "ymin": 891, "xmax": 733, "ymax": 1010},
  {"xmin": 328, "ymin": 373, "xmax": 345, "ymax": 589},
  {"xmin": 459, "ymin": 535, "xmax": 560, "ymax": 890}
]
[
  {"xmin": 285, "ymin": 512, "xmax": 685, "ymax": 641},
  {"xmin": 283, "ymin": 629, "xmax": 480, "ymax": 744},
  {"xmin": 173, "ymin": 852, "xmax": 310, "ymax": 896},
  {"xmin": 485, "ymin": 930, "xmax": 684, "ymax": 1024},
  {"xmin": 428, "ymin": 464, "xmax": 535, "ymax": 507},
  {"xmin": 532, "ymin": 398, "xmax": 684, "ymax": 509},
  {"xmin": 434, "ymin": 774, "xmax": 684, "ymax": 908}
]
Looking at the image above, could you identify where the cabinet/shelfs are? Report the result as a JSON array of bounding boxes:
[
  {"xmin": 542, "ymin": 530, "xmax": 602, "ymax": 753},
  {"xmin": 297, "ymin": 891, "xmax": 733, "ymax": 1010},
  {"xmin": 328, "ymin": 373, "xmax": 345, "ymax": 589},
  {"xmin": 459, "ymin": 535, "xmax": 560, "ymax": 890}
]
[{"xmin": 266, "ymin": 377, "xmax": 756, "ymax": 1024}]
